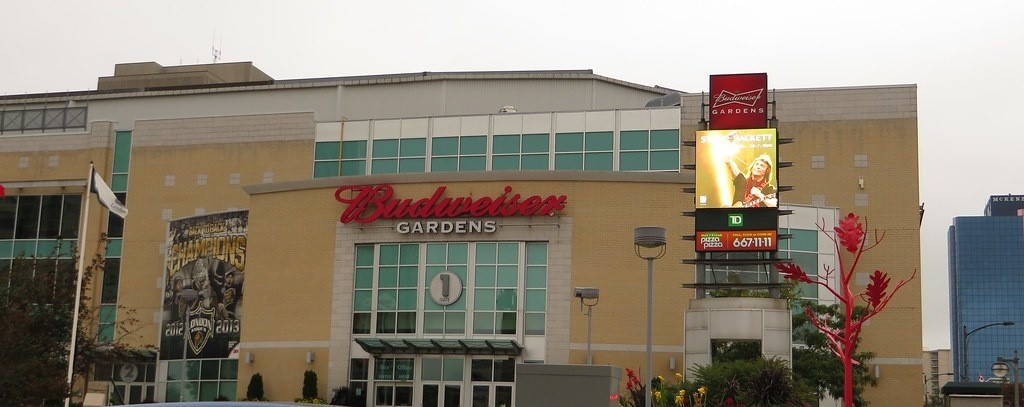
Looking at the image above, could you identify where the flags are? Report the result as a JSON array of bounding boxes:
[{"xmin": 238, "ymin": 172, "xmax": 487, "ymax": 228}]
[{"xmin": 90, "ymin": 166, "xmax": 129, "ymax": 218}]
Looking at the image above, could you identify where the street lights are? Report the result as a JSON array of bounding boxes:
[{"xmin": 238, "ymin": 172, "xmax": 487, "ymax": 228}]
[
  {"xmin": 923, "ymin": 371, "xmax": 957, "ymax": 407},
  {"xmin": 962, "ymin": 318, "xmax": 1016, "ymax": 381}
]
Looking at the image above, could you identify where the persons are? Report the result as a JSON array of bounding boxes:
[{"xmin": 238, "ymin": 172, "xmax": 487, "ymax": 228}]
[
  {"xmin": 214, "ymin": 268, "xmax": 237, "ymax": 320},
  {"xmin": 163, "ymin": 276, "xmax": 189, "ymax": 321},
  {"xmin": 721, "ymin": 132, "xmax": 777, "ymax": 207}
]
